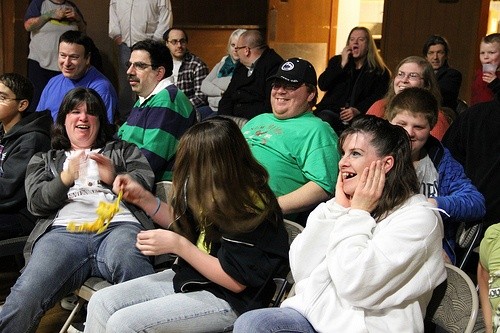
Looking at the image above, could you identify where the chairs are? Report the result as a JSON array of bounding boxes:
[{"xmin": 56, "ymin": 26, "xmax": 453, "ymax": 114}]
[{"xmin": 59, "ymin": 181, "xmax": 493, "ymax": 333}]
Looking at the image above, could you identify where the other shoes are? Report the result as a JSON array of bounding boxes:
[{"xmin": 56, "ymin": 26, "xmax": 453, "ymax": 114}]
[{"xmin": 60, "ymin": 296, "xmax": 78, "ymax": 311}]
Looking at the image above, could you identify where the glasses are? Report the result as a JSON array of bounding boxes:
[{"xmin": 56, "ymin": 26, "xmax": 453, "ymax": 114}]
[
  {"xmin": 235, "ymin": 46, "xmax": 250, "ymax": 52},
  {"xmin": 167, "ymin": 39, "xmax": 187, "ymax": 45},
  {"xmin": 128, "ymin": 61, "xmax": 162, "ymax": 70},
  {"xmin": 0, "ymin": 96, "xmax": 24, "ymax": 103},
  {"xmin": 396, "ymin": 71, "xmax": 426, "ymax": 80}
]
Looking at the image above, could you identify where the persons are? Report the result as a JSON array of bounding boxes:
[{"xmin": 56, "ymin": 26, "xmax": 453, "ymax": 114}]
[
  {"xmin": 240, "ymin": 57, "xmax": 343, "ymax": 222},
  {"xmin": 470, "ymin": 33, "xmax": 500, "ymax": 105},
  {"xmin": 36, "ymin": 30, "xmax": 117, "ymax": 123},
  {"xmin": 0, "ymin": 86, "xmax": 156, "ymax": 333},
  {"xmin": 366, "ymin": 56, "xmax": 450, "ymax": 142},
  {"xmin": 313, "ymin": 27, "xmax": 392, "ymax": 136},
  {"xmin": 163, "ymin": 27, "xmax": 208, "ymax": 109},
  {"xmin": 198, "ymin": 29, "xmax": 247, "ymax": 121},
  {"xmin": 422, "ymin": 36, "xmax": 462, "ymax": 112},
  {"xmin": 109, "ymin": 0, "xmax": 173, "ymax": 127},
  {"xmin": 24, "ymin": 0, "xmax": 87, "ymax": 111},
  {"xmin": 233, "ymin": 114, "xmax": 448, "ymax": 333},
  {"xmin": 217, "ymin": 30, "xmax": 285, "ymax": 119},
  {"xmin": 0, "ymin": 73, "xmax": 55, "ymax": 240},
  {"xmin": 384, "ymin": 87, "xmax": 486, "ymax": 264},
  {"xmin": 112, "ymin": 39, "xmax": 197, "ymax": 195},
  {"xmin": 84, "ymin": 116, "xmax": 290, "ymax": 333}
]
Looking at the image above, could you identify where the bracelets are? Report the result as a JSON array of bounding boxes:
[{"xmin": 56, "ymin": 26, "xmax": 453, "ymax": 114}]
[{"xmin": 146, "ymin": 197, "xmax": 160, "ymax": 216}]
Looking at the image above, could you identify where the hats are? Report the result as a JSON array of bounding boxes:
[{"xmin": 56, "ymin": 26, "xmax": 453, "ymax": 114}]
[{"xmin": 268, "ymin": 58, "xmax": 317, "ymax": 88}]
